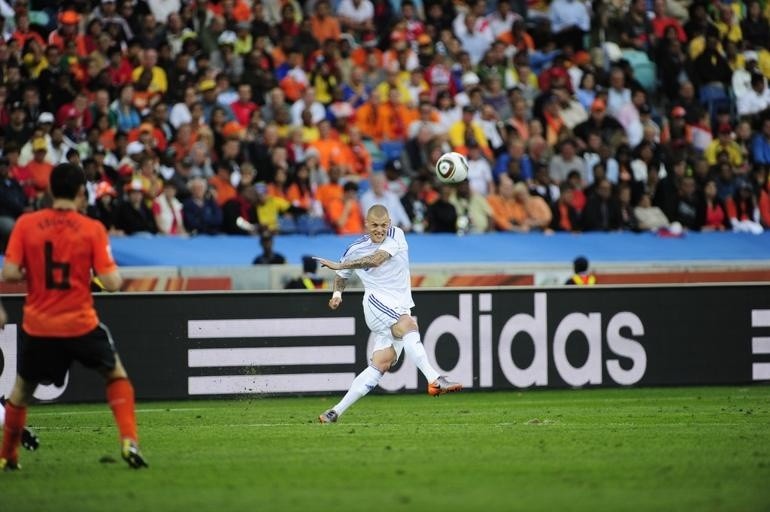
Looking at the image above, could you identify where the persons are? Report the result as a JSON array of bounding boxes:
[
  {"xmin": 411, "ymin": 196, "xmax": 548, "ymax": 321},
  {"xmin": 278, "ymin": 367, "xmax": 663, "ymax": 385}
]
[
  {"xmin": 560, "ymin": 255, "xmax": 596, "ymax": 287},
  {"xmin": 0, "ymin": 0, "xmax": 770, "ymax": 246},
  {"xmin": 249, "ymin": 228, "xmax": 286, "ymax": 264},
  {"xmin": 284, "ymin": 253, "xmax": 326, "ymax": 291},
  {"xmin": 307, "ymin": 202, "xmax": 463, "ymax": 424},
  {"xmin": 0, "ymin": 163, "xmax": 153, "ymax": 469}
]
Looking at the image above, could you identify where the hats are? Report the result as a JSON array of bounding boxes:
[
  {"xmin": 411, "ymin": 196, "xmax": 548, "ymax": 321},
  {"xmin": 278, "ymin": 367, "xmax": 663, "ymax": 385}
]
[{"xmin": 124, "ymin": 139, "xmax": 146, "ymax": 155}]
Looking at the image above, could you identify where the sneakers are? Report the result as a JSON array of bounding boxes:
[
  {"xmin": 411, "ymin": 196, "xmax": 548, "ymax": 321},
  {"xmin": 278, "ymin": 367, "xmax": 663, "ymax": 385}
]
[
  {"xmin": 20, "ymin": 428, "xmax": 41, "ymax": 453},
  {"xmin": 0, "ymin": 458, "xmax": 23, "ymax": 473},
  {"xmin": 317, "ymin": 408, "xmax": 338, "ymax": 425},
  {"xmin": 427, "ymin": 375, "xmax": 464, "ymax": 398},
  {"xmin": 120, "ymin": 437, "xmax": 150, "ymax": 471}
]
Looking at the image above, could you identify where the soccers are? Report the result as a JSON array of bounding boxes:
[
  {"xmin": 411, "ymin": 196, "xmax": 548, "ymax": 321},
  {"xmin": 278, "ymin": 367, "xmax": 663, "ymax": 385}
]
[{"xmin": 435, "ymin": 152, "xmax": 469, "ymax": 184}]
